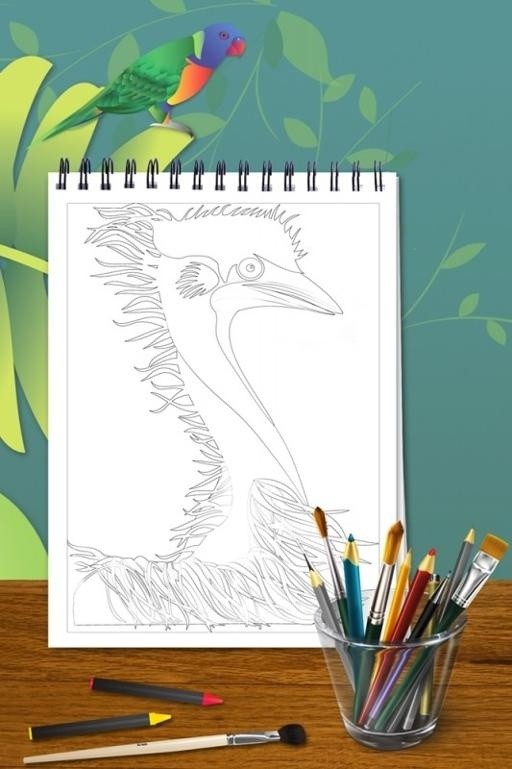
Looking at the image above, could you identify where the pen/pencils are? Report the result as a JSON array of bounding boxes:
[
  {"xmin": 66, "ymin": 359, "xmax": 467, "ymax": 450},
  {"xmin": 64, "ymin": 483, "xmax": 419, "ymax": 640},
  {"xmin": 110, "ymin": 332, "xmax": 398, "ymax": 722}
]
[
  {"xmin": 303, "ymin": 505, "xmax": 509, "ymax": 734},
  {"xmin": 86, "ymin": 677, "xmax": 227, "ymax": 708},
  {"xmin": 26, "ymin": 710, "xmax": 177, "ymax": 742}
]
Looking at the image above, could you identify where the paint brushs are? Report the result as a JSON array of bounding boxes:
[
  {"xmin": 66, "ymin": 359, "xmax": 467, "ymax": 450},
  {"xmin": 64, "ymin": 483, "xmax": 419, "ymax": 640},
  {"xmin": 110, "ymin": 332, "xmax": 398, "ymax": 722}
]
[{"xmin": 22, "ymin": 722, "xmax": 308, "ymax": 767}]
